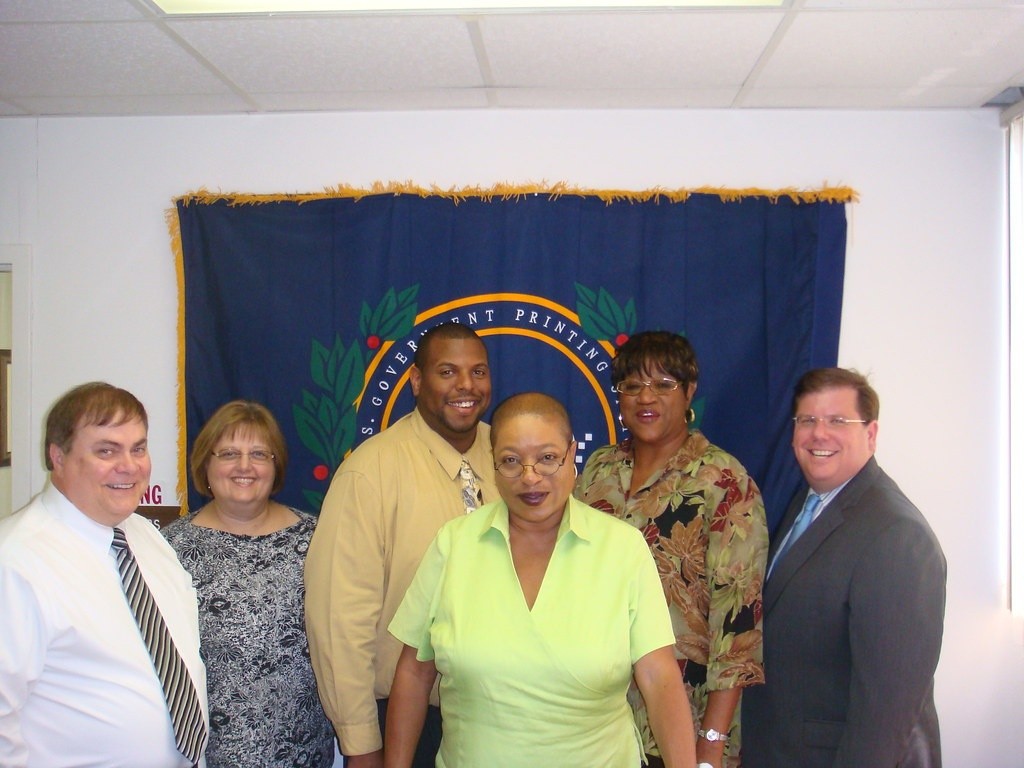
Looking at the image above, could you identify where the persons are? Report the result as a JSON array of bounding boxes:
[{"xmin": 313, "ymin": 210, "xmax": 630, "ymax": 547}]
[
  {"xmin": 741, "ymin": 367, "xmax": 947, "ymax": 768},
  {"xmin": 0, "ymin": 382, "xmax": 210, "ymax": 768},
  {"xmin": 303, "ymin": 324, "xmax": 509, "ymax": 768},
  {"xmin": 382, "ymin": 393, "xmax": 697, "ymax": 768},
  {"xmin": 157, "ymin": 399, "xmax": 336, "ymax": 768},
  {"xmin": 574, "ymin": 330, "xmax": 770, "ymax": 768}
]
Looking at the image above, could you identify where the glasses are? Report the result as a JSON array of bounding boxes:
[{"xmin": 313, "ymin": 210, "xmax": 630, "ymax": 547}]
[
  {"xmin": 792, "ymin": 414, "xmax": 868, "ymax": 430},
  {"xmin": 211, "ymin": 447, "xmax": 275, "ymax": 465},
  {"xmin": 616, "ymin": 379, "xmax": 684, "ymax": 395},
  {"xmin": 494, "ymin": 440, "xmax": 570, "ymax": 479}
]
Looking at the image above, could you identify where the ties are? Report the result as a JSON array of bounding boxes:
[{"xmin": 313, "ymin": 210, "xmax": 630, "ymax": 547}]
[
  {"xmin": 765, "ymin": 494, "xmax": 820, "ymax": 582},
  {"xmin": 460, "ymin": 457, "xmax": 478, "ymax": 514},
  {"xmin": 110, "ymin": 526, "xmax": 208, "ymax": 766}
]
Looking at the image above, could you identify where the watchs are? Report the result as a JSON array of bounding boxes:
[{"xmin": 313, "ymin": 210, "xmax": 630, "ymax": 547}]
[{"xmin": 697, "ymin": 728, "xmax": 729, "ymax": 743}]
